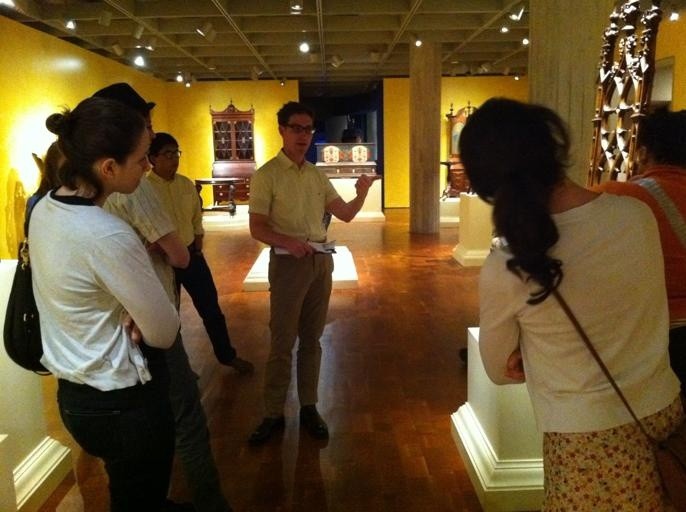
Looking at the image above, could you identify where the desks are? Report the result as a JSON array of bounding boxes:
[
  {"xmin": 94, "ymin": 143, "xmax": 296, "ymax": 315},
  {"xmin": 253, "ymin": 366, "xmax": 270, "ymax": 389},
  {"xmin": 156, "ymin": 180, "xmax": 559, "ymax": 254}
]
[
  {"xmin": 440, "ymin": 161, "xmax": 476, "ymax": 201},
  {"xmin": 194, "ymin": 177, "xmax": 240, "ymax": 216}
]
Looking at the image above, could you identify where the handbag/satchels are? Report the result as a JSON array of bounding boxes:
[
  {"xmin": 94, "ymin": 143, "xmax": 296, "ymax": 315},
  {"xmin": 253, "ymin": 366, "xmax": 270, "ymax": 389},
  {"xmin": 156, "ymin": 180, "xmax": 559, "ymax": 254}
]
[
  {"xmin": 3, "ymin": 195, "xmax": 50, "ymax": 372},
  {"xmin": 654, "ymin": 420, "xmax": 686, "ymax": 512}
]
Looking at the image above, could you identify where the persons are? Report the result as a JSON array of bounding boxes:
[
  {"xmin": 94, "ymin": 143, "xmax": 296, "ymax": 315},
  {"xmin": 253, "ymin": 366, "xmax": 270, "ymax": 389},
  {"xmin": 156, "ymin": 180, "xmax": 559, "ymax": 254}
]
[
  {"xmin": 590, "ymin": 110, "xmax": 684, "ymax": 405},
  {"xmin": 147, "ymin": 132, "xmax": 253, "ymax": 373},
  {"xmin": 457, "ymin": 95, "xmax": 684, "ymax": 511},
  {"xmin": 93, "ymin": 82, "xmax": 231, "ymax": 512},
  {"xmin": 26, "ymin": 142, "xmax": 67, "ymax": 228},
  {"xmin": 28, "ymin": 97, "xmax": 181, "ymax": 511},
  {"xmin": 248, "ymin": 101, "xmax": 382, "ymax": 446}
]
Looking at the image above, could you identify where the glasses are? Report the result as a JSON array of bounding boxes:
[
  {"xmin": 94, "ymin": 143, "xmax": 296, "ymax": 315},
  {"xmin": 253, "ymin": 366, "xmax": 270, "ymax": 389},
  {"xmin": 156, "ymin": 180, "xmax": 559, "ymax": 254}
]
[
  {"xmin": 282, "ymin": 124, "xmax": 317, "ymax": 135},
  {"xmin": 157, "ymin": 151, "xmax": 181, "ymax": 159}
]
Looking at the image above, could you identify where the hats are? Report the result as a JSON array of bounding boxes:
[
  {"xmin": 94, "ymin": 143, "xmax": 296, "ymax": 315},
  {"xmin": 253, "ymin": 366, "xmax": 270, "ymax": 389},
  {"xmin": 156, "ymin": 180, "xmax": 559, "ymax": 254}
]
[{"xmin": 89, "ymin": 82, "xmax": 157, "ymax": 114}]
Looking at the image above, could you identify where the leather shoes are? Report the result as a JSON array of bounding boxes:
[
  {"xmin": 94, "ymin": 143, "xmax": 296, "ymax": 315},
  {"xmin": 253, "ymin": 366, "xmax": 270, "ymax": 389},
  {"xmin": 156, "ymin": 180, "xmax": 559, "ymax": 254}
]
[
  {"xmin": 224, "ymin": 356, "xmax": 254, "ymax": 373},
  {"xmin": 301, "ymin": 405, "xmax": 328, "ymax": 439},
  {"xmin": 248, "ymin": 417, "xmax": 285, "ymax": 447}
]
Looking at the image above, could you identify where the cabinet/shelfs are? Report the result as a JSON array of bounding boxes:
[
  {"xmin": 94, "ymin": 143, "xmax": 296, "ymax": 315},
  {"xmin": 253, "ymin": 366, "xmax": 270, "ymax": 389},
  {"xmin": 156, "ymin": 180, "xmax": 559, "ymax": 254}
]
[
  {"xmin": 209, "ymin": 99, "xmax": 257, "ymax": 206},
  {"xmin": 446, "ymin": 100, "xmax": 479, "ymax": 197}
]
